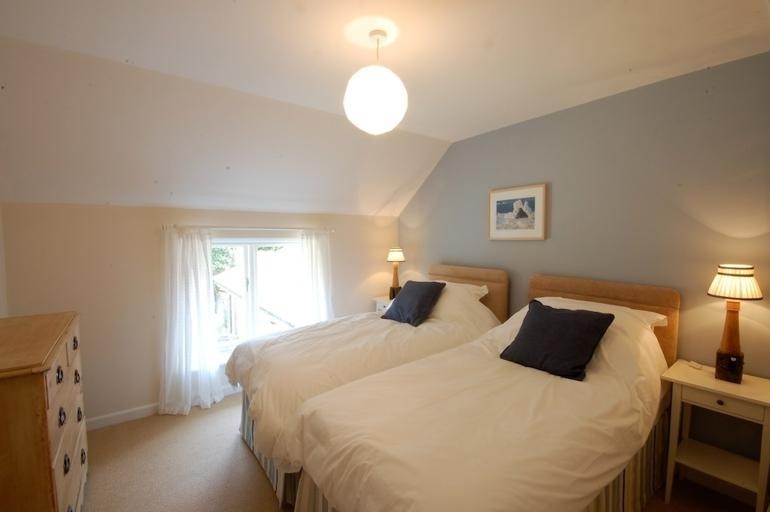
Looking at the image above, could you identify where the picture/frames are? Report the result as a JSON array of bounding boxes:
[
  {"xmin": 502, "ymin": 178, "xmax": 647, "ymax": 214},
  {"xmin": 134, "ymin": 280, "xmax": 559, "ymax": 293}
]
[{"xmin": 489, "ymin": 183, "xmax": 551, "ymax": 241}]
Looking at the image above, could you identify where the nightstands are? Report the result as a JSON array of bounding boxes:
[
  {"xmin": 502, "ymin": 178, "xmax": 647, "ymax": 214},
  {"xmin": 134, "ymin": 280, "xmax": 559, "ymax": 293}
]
[
  {"xmin": 373, "ymin": 293, "xmax": 392, "ymax": 311},
  {"xmin": 663, "ymin": 359, "xmax": 770, "ymax": 512}
]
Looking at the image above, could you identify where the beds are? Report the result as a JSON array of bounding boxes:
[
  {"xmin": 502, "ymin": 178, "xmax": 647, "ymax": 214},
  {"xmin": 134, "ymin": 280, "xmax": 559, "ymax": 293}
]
[
  {"xmin": 273, "ymin": 273, "xmax": 680, "ymax": 512},
  {"xmin": 224, "ymin": 263, "xmax": 511, "ymax": 512}
]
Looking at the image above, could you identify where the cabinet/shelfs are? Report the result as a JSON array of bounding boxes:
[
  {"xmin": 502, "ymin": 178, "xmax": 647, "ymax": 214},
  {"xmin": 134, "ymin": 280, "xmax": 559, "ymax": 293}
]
[{"xmin": 0, "ymin": 310, "xmax": 89, "ymax": 512}]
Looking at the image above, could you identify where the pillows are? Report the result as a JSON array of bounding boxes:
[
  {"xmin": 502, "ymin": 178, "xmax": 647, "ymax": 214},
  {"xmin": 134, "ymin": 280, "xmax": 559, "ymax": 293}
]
[
  {"xmin": 382, "ymin": 280, "xmax": 445, "ymax": 327},
  {"xmin": 484, "ymin": 297, "xmax": 668, "ymax": 372},
  {"xmin": 498, "ymin": 299, "xmax": 616, "ymax": 380},
  {"xmin": 414, "ymin": 276, "xmax": 488, "ymax": 320}
]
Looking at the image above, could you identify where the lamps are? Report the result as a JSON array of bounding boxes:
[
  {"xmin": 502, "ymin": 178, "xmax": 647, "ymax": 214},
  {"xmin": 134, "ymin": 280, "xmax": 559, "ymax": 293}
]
[
  {"xmin": 704, "ymin": 264, "xmax": 764, "ymax": 383},
  {"xmin": 342, "ymin": 31, "xmax": 409, "ymax": 136},
  {"xmin": 387, "ymin": 247, "xmax": 406, "ymax": 300}
]
